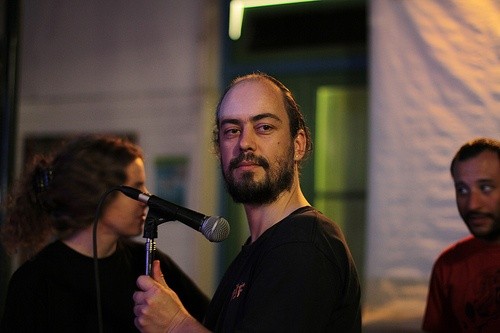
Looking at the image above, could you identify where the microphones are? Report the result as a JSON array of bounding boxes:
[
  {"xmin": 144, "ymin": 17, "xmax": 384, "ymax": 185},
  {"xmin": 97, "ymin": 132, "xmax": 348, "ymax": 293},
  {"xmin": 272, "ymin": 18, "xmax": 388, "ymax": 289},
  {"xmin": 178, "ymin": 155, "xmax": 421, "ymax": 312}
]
[{"xmin": 119, "ymin": 185, "xmax": 229, "ymax": 242}]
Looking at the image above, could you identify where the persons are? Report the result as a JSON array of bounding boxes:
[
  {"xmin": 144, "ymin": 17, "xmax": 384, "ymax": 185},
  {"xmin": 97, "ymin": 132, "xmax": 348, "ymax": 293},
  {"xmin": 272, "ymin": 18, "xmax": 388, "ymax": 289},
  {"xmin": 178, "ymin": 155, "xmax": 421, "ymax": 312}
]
[
  {"xmin": 1, "ymin": 131, "xmax": 211, "ymax": 333},
  {"xmin": 132, "ymin": 73, "xmax": 363, "ymax": 333},
  {"xmin": 422, "ymin": 137, "xmax": 500, "ymax": 333}
]
